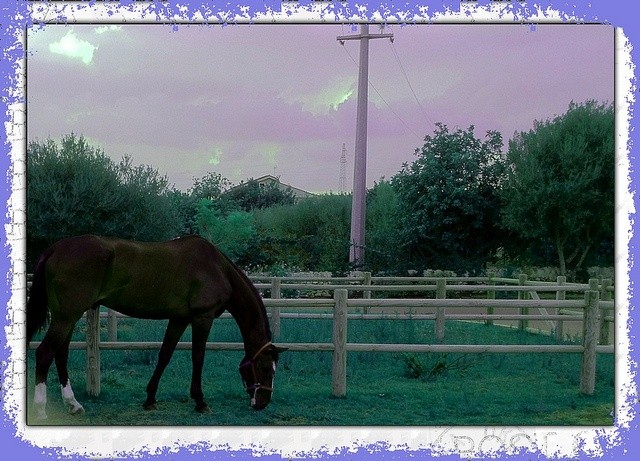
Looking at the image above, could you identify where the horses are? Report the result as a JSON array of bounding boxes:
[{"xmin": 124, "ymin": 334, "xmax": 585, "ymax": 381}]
[{"xmin": 28, "ymin": 234, "xmax": 289, "ymax": 424}]
